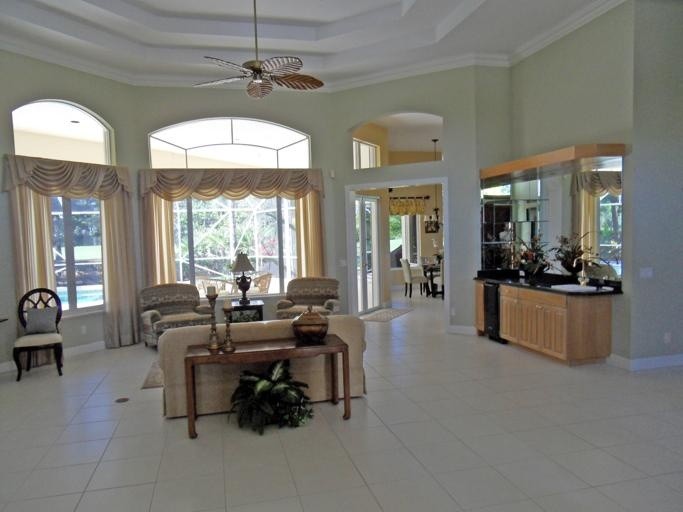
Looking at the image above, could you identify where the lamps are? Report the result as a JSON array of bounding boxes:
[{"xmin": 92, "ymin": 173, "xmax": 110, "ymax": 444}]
[
  {"xmin": 423, "ymin": 212, "xmax": 437, "ymax": 232},
  {"xmin": 229, "ymin": 253, "xmax": 254, "ymax": 304}
]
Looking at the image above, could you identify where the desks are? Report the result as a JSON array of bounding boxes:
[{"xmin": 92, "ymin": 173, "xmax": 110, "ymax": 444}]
[
  {"xmin": 209, "ymin": 275, "xmax": 247, "ymax": 295},
  {"xmin": 421, "ymin": 262, "xmax": 441, "ymax": 275},
  {"xmin": 183, "ymin": 334, "xmax": 350, "ymax": 432},
  {"xmin": 222, "ymin": 300, "xmax": 265, "ymax": 322}
]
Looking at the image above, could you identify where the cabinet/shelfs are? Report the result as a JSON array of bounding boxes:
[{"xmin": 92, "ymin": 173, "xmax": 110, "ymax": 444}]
[
  {"xmin": 472, "ymin": 278, "xmax": 613, "ymax": 367},
  {"xmin": 480, "ymin": 197, "xmax": 548, "ymax": 270}
]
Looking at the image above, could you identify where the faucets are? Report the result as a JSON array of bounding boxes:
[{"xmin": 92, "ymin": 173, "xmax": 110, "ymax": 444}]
[{"xmin": 572, "ymin": 257, "xmax": 590, "ymax": 286}]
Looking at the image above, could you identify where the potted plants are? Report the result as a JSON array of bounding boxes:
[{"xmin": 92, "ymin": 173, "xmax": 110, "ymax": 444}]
[{"xmin": 227, "ymin": 360, "xmax": 313, "ymax": 431}]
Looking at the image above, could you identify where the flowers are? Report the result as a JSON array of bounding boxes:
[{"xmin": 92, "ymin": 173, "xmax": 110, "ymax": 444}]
[
  {"xmin": 510, "ymin": 237, "xmax": 562, "ymax": 275},
  {"xmin": 546, "ymin": 233, "xmax": 600, "ymax": 261}
]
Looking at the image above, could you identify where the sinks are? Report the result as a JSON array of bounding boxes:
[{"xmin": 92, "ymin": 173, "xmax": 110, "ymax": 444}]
[{"xmin": 550, "ymin": 283, "xmax": 614, "ymax": 293}]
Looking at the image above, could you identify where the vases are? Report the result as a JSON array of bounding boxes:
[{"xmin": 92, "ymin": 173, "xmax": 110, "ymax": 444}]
[
  {"xmin": 562, "ymin": 260, "xmax": 582, "ymax": 277},
  {"xmin": 523, "ymin": 271, "xmax": 554, "ymax": 288}
]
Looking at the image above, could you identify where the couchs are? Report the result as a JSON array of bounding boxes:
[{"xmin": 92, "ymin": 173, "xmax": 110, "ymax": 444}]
[
  {"xmin": 136, "ymin": 283, "xmax": 215, "ymax": 349},
  {"xmin": 274, "ymin": 276, "xmax": 342, "ymax": 319},
  {"xmin": 157, "ymin": 313, "xmax": 367, "ymax": 418}
]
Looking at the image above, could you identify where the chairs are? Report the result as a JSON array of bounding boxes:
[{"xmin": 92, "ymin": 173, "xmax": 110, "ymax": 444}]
[
  {"xmin": 399, "ymin": 257, "xmax": 426, "ymax": 298},
  {"xmin": 431, "ymin": 259, "xmax": 443, "ymax": 300},
  {"xmin": 252, "ymin": 273, "xmax": 273, "ymax": 294},
  {"xmin": 10, "ymin": 287, "xmax": 64, "ymax": 382}
]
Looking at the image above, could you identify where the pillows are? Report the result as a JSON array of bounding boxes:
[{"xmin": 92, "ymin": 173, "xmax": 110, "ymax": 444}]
[{"xmin": 25, "ymin": 307, "xmax": 58, "ymax": 333}]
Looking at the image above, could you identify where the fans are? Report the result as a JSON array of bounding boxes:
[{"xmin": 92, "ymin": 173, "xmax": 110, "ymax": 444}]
[{"xmin": 195, "ymin": 1, "xmax": 323, "ymax": 97}]
